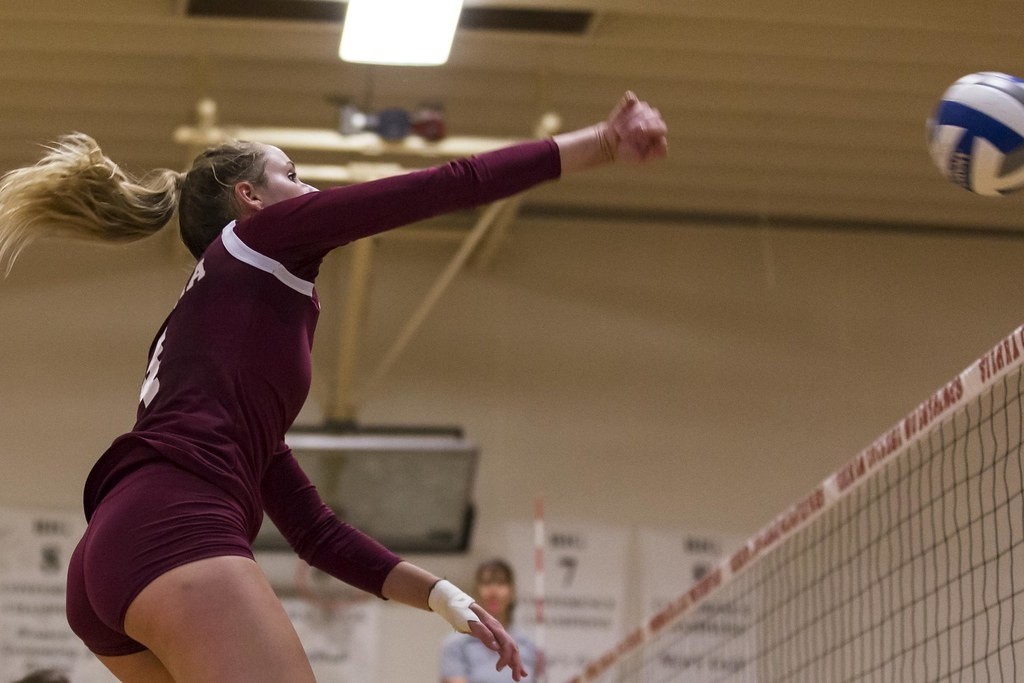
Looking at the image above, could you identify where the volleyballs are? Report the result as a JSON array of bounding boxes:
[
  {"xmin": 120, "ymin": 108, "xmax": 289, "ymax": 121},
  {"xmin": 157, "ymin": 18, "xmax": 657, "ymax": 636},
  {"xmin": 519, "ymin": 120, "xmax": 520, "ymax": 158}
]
[{"xmin": 924, "ymin": 71, "xmax": 1023, "ymax": 196}]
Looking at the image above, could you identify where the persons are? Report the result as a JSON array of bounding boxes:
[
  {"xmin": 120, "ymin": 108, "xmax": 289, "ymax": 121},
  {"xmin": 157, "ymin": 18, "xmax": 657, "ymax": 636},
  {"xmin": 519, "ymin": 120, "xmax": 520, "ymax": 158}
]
[{"xmin": 0, "ymin": 91, "xmax": 667, "ymax": 683}]
